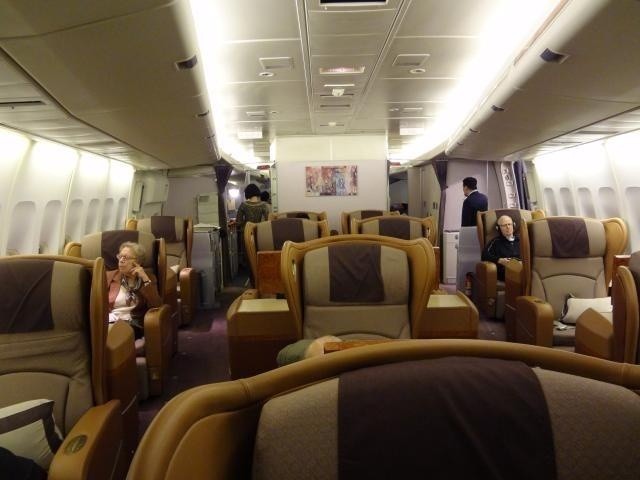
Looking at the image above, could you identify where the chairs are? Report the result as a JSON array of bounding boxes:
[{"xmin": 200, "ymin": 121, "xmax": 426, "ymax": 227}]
[{"xmin": 0, "ymin": 207, "xmax": 639, "ymax": 478}]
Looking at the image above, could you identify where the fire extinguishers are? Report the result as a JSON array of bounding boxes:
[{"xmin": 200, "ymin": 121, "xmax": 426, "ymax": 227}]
[{"xmin": 465, "ymin": 272, "xmax": 477, "ymax": 301}]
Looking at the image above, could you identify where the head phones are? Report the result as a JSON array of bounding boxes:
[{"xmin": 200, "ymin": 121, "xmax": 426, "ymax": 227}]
[{"xmin": 496, "ymin": 222, "xmax": 517, "ymax": 232}]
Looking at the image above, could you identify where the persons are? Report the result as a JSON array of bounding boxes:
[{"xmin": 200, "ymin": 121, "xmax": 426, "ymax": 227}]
[
  {"xmin": 231, "ymin": 184, "xmax": 271, "ymax": 226},
  {"xmin": 261, "ymin": 192, "xmax": 272, "ymax": 207},
  {"xmin": 485, "ymin": 214, "xmax": 520, "ymax": 278},
  {"xmin": 100, "ymin": 242, "xmax": 161, "ymax": 326},
  {"xmin": 461, "ymin": 178, "xmax": 487, "ymax": 227}
]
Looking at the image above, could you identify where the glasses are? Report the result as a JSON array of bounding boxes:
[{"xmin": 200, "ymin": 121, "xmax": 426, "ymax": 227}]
[
  {"xmin": 499, "ymin": 223, "xmax": 514, "ymax": 226},
  {"xmin": 115, "ymin": 254, "xmax": 138, "ymax": 261}
]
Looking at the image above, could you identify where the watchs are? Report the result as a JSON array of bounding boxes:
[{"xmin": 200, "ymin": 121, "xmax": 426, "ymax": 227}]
[{"xmin": 144, "ymin": 280, "xmax": 152, "ymax": 284}]
